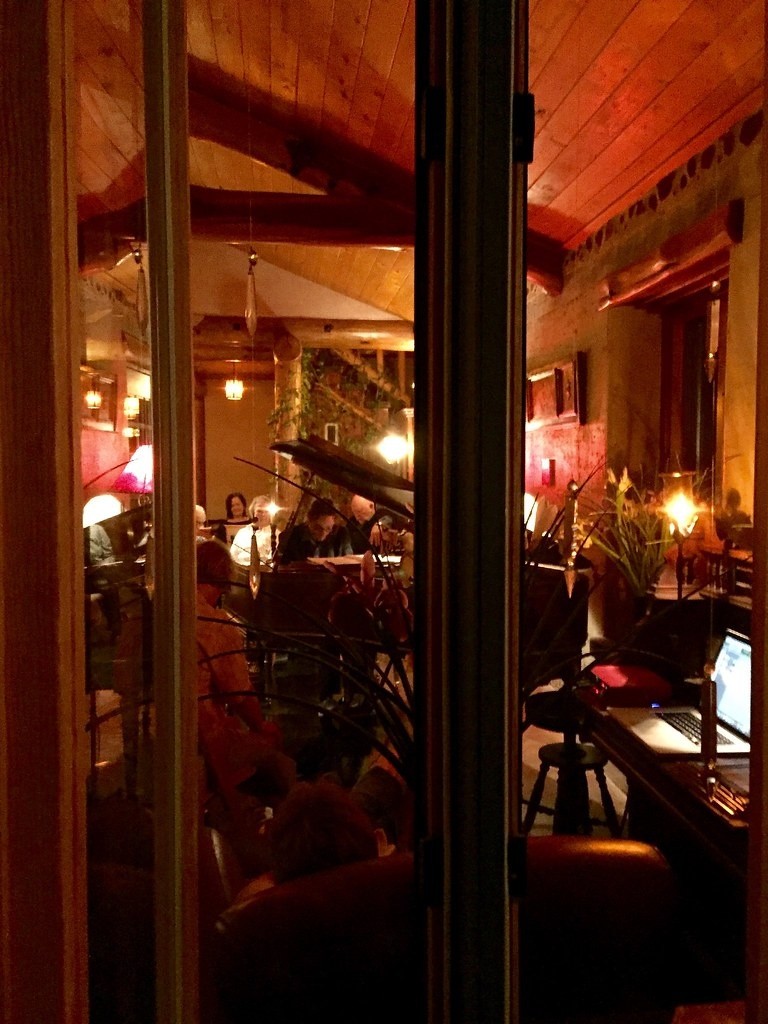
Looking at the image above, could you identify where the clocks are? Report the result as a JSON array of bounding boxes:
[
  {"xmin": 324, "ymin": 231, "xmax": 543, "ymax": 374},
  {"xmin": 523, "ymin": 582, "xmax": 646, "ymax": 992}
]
[{"xmin": 273, "ymin": 333, "xmax": 301, "ymax": 362}]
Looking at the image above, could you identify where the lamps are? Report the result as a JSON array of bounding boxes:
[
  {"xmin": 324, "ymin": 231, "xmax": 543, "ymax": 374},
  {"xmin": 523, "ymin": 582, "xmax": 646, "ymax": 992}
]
[
  {"xmin": 84, "ymin": 375, "xmax": 102, "ymax": 409},
  {"xmin": 223, "ymin": 360, "xmax": 244, "ymax": 400},
  {"xmin": 105, "ymin": 444, "xmax": 154, "ymax": 506},
  {"xmin": 123, "ymin": 380, "xmax": 139, "ymax": 420}
]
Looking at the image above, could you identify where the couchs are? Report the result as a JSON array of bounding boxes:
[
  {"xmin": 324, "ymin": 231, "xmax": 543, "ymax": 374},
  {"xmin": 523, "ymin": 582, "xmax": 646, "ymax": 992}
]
[{"xmin": 209, "ymin": 839, "xmax": 668, "ymax": 1024}]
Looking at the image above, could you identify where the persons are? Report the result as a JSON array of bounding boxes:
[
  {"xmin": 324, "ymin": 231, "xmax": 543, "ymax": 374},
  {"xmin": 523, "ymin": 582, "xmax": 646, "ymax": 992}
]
[
  {"xmin": 278, "ymin": 499, "xmax": 352, "ymax": 703},
  {"xmin": 233, "ymin": 784, "xmax": 399, "ymax": 906},
  {"xmin": 193, "ymin": 541, "xmax": 296, "ymax": 786},
  {"xmin": 346, "ymin": 495, "xmax": 375, "ymax": 554},
  {"xmin": 229, "ymin": 496, "xmax": 281, "ymax": 566},
  {"xmin": 196, "ymin": 505, "xmax": 207, "ymax": 543},
  {"xmin": 214, "ymin": 493, "xmax": 252, "ymax": 544}
]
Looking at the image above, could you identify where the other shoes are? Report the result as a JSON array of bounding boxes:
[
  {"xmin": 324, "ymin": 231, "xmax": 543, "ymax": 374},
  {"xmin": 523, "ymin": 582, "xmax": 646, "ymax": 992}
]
[
  {"xmin": 350, "ymin": 694, "xmax": 365, "ymax": 708},
  {"xmin": 318, "ymin": 688, "xmax": 344, "ymax": 715}
]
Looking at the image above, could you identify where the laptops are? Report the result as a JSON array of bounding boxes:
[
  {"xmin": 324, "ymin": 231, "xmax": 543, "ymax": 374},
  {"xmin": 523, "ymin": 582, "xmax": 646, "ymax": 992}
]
[{"xmin": 607, "ymin": 628, "xmax": 752, "ymax": 758}]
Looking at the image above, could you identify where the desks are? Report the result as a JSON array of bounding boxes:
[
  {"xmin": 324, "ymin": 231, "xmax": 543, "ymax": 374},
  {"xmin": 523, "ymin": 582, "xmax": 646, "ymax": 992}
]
[{"xmin": 576, "ymin": 685, "xmax": 749, "ymax": 885}]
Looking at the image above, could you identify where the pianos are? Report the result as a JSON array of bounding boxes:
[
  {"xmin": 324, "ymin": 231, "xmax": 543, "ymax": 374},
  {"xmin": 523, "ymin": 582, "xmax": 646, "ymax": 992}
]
[{"xmin": 219, "ymin": 433, "xmax": 594, "ymax": 746}]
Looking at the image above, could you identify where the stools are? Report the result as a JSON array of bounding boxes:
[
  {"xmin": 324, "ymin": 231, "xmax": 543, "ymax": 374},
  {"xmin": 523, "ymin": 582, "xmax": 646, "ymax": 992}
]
[{"xmin": 521, "ymin": 743, "xmax": 622, "ymax": 838}]
